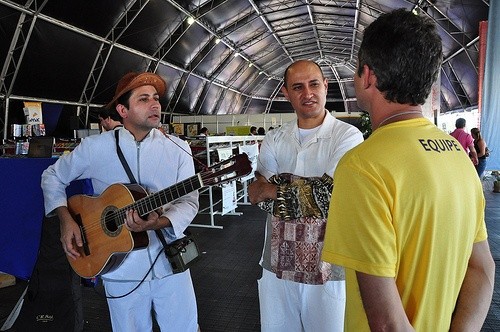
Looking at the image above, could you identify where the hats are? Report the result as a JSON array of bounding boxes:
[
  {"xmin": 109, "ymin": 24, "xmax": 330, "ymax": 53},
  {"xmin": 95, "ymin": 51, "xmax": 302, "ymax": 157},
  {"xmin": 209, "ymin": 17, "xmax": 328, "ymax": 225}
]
[{"xmin": 105, "ymin": 72, "xmax": 167, "ymax": 109}]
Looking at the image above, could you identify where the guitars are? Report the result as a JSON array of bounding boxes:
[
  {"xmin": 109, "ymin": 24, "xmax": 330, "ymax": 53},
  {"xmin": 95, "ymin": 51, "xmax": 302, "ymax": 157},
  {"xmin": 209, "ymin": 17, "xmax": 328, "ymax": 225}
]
[{"xmin": 63, "ymin": 152, "xmax": 253, "ymax": 280}]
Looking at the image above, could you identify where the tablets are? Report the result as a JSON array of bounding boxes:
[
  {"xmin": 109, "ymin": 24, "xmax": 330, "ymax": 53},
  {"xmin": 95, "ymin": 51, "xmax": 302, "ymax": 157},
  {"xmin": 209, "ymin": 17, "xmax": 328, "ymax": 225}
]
[{"xmin": 28, "ymin": 136, "xmax": 53, "ymax": 159}]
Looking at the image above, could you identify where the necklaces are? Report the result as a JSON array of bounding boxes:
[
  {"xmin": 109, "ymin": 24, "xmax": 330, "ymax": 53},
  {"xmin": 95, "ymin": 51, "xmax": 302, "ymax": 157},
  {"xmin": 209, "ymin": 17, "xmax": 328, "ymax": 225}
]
[{"xmin": 375, "ymin": 110, "xmax": 423, "ymax": 129}]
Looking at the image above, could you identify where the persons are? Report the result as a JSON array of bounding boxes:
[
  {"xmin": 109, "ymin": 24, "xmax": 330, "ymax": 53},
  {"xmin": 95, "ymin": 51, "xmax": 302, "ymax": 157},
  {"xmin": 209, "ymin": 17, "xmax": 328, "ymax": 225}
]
[
  {"xmin": 449, "ymin": 118, "xmax": 479, "ymax": 165},
  {"xmin": 41, "ymin": 72, "xmax": 200, "ymax": 332},
  {"xmin": 248, "ymin": 126, "xmax": 275, "ymax": 136},
  {"xmin": 469, "ymin": 128, "xmax": 486, "ymax": 177},
  {"xmin": 200, "ymin": 127, "xmax": 209, "ymax": 136},
  {"xmin": 248, "ymin": 59, "xmax": 365, "ymax": 332},
  {"xmin": 322, "ymin": 7, "xmax": 495, "ymax": 332},
  {"xmin": 97, "ymin": 104, "xmax": 124, "ymax": 131}
]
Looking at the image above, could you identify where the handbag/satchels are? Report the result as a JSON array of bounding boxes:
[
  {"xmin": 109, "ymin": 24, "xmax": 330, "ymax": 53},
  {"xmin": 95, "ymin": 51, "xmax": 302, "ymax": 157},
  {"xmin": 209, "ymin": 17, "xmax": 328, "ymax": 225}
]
[
  {"xmin": 164, "ymin": 236, "xmax": 202, "ymax": 274},
  {"xmin": 483, "ymin": 147, "xmax": 490, "ymax": 157}
]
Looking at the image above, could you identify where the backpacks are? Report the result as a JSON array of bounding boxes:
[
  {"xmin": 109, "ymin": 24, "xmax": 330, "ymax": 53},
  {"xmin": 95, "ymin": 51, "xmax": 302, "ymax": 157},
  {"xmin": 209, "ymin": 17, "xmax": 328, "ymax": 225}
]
[{"xmin": 480, "ymin": 170, "xmax": 500, "ymax": 193}]
